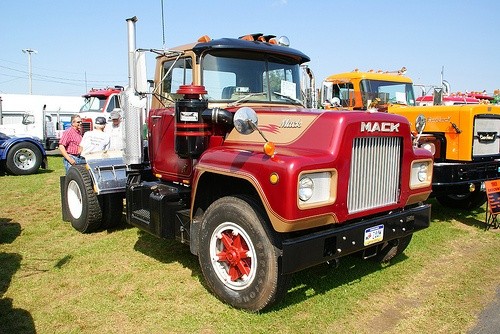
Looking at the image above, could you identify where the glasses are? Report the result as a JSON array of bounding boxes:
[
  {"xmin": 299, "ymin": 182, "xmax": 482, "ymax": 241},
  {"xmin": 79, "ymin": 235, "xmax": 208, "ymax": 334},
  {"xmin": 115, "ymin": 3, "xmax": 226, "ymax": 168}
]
[{"xmin": 77, "ymin": 121, "xmax": 82, "ymax": 124}]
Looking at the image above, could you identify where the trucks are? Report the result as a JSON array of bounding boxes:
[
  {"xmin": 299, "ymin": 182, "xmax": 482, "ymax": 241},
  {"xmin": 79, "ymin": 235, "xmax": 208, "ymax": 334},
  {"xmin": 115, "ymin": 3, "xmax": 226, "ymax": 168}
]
[{"xmin": 1, "ymin": 105, "xmax": 64, "ymax": 153}]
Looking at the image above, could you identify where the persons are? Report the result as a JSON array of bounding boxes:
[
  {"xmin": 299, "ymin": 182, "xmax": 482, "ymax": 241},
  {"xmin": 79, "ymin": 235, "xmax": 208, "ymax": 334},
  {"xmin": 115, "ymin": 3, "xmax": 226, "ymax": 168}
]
[{"xmin": 59, "ymin": 111, "xmax": 125, "ymax": 175}]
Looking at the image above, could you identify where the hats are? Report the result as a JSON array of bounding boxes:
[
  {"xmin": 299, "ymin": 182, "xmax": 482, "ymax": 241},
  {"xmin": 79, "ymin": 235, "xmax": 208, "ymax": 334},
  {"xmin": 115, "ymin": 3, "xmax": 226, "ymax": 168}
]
[
  {"xmin": 111, "ymin": 111, "xmax": 120, "ymax": 120},
  {"xmin": 95, "ymin": 117, "xmax": 108, "ymax": 125}
]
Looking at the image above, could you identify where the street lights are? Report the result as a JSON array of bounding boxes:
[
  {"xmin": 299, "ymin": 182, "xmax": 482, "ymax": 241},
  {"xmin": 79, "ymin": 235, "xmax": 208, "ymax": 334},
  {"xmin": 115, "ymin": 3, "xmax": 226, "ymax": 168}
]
[{"xmin": 22, "ymin": 48, "xmax": 38, "ymax": 94}]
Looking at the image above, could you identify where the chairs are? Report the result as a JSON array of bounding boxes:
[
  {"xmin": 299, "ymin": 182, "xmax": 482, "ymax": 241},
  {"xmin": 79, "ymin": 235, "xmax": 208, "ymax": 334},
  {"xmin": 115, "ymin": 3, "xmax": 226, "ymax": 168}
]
[{"xmin": 221, "ymin": 86, "xmax": 238, "ymax": 98}]
